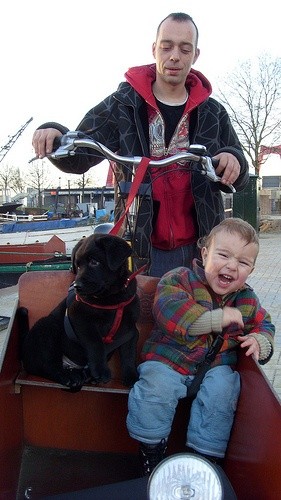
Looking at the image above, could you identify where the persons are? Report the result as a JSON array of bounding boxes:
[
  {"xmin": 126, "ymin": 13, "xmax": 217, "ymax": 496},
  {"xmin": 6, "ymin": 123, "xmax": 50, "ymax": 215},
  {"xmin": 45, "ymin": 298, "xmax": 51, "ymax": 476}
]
[
  {"xmin": 119, "ymin": 215, "xmax": 275, "ymax": 488},
  {"xmin": 34, "ymin": 11, "xmax": 251, "ymax": 277}
]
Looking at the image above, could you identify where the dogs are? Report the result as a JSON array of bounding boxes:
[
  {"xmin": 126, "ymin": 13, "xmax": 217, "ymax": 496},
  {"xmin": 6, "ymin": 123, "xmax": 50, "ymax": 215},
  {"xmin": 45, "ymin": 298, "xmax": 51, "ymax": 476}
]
[{"xmin": 17, "ymin": 234, "xmax": 141, "ymax": 391}]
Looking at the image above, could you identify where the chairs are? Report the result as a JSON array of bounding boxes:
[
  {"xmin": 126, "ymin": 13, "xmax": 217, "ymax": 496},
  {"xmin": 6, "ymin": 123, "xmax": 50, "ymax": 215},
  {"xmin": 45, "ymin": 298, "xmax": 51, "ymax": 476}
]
[{"xmin": 0, "ymin": 266, "xmax": 281, "ymax": 500}]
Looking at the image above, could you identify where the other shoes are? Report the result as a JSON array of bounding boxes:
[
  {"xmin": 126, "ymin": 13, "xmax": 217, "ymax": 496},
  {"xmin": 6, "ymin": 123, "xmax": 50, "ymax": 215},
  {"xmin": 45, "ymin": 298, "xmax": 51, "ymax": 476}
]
[{"xmin": 140, "ymin": 438, "xmax": 173, "ymax": 477}]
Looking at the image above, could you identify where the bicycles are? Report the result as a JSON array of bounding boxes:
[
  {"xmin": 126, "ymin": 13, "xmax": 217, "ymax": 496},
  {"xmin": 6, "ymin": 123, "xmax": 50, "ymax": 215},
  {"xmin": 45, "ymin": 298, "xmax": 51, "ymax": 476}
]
[{"xmin": 28, "ymin": 124, "xmax": 236, "ymax": 271}]
